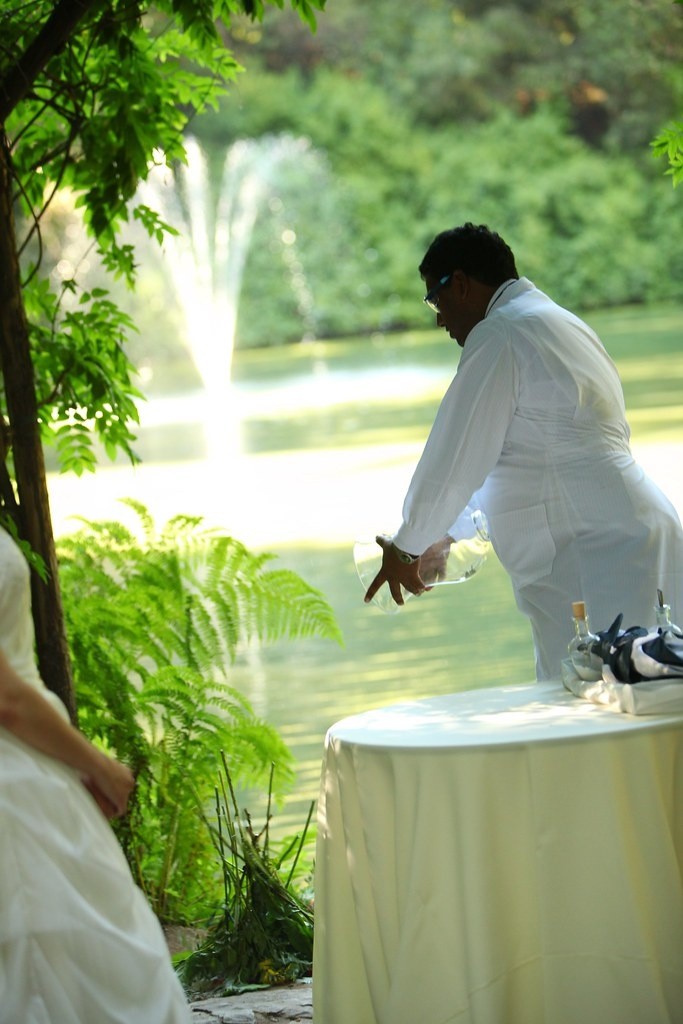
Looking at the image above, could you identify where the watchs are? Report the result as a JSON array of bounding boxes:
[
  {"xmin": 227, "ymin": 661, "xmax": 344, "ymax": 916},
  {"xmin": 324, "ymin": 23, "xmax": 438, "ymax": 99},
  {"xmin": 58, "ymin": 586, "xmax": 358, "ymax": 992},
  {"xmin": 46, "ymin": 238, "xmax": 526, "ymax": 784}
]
[{"xmin": 391, "ymin": 543, "xmax": 419, "ymax": 565}]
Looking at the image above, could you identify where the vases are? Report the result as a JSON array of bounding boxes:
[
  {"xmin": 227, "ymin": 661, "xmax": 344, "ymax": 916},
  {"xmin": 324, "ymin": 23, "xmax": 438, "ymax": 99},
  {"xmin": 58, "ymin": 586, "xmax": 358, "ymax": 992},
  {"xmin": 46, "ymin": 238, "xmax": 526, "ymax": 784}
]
[{"xmin": 353, "ymin": 504, "xmax": 492, "ymax": 615}]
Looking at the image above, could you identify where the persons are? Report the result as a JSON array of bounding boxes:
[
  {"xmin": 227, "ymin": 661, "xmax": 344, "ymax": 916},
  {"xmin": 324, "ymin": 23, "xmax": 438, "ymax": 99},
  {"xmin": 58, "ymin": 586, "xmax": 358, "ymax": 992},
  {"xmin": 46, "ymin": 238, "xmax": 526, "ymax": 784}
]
[
  {"xmin": 363, "ymin": 221, "xmax": 682, "ymax": 683},
  {"xmin": 0, "ymin": 525, "xmax": 198, "ymax": 1024}
]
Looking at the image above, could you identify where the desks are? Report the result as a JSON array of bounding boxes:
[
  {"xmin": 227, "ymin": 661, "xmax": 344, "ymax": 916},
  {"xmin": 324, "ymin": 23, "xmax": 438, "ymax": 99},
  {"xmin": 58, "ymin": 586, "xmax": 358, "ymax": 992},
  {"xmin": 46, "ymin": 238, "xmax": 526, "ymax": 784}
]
[{"xmin": 312, "ymin": 678, "xmax": 683, "ymax": 1024}]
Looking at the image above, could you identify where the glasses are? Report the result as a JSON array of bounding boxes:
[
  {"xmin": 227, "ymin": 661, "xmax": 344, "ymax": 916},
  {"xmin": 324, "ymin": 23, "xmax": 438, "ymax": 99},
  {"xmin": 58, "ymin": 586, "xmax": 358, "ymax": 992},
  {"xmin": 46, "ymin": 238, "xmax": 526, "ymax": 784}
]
[{"xmin": 422, "ymin": 274, "xmax": 453, "ymax": 313}]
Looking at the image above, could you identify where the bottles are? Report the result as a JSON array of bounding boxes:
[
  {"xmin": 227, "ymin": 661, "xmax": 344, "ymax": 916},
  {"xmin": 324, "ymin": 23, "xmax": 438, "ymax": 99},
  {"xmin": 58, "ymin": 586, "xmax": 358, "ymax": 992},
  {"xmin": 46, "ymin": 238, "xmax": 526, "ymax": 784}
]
[
  {"xmin": 568, "ymin": 602, "xmax": 604, "ymax": 681},
  {"xmin": 352, "ymin": 506, "xmax": 490, "ymax": 614},
  {"xmin": 650, "ymin": 605, "xmax": 683, "ymax": 636}
]
